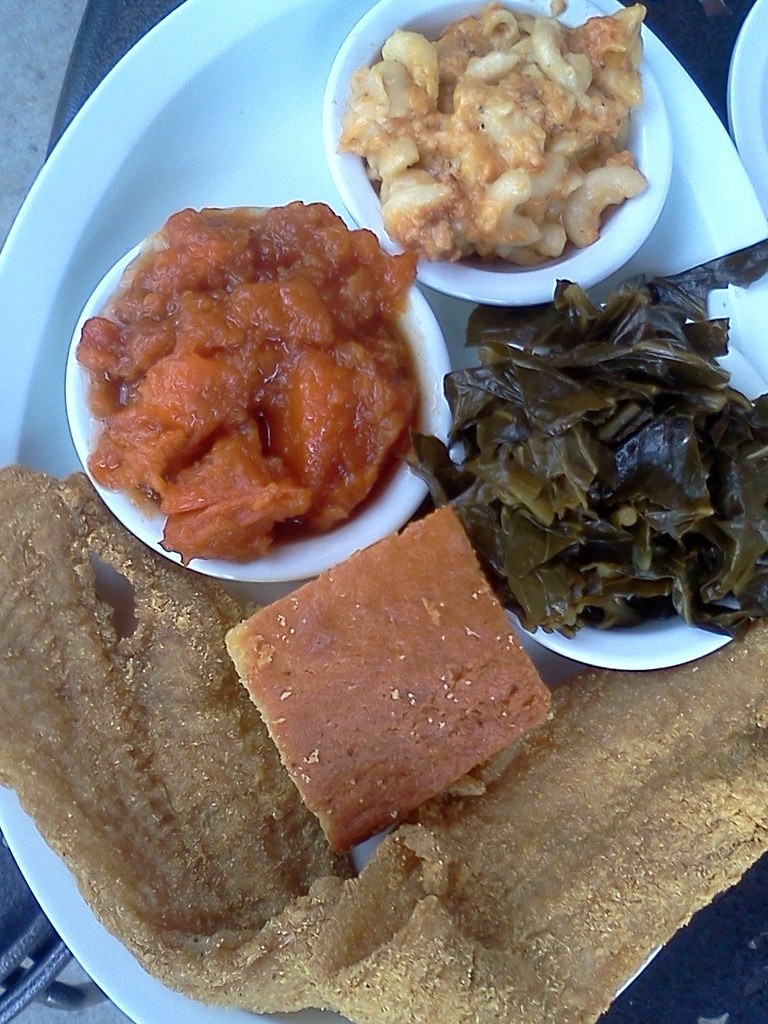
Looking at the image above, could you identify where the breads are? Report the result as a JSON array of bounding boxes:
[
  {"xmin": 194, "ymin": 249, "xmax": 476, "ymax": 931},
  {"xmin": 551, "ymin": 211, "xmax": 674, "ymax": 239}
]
[{"xmin": 225, "ymin": 505, "xmax": 555, "ymax": 855}]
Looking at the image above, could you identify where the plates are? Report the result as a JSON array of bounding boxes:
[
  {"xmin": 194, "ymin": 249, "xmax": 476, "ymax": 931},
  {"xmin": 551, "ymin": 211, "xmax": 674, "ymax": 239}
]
[{"xmin": 0, "ymin": 0, "xmax": 768, "ymax": 1024}]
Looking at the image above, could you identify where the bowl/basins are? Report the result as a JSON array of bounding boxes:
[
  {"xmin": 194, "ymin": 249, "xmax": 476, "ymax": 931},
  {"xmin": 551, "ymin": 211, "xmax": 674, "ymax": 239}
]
[
  {"xmin": 66, "ymin": 230, "xmax": 451, "ymax": 583},
  {"xmin": 448, "ymin": 319, "xmax": 768, "ymax": 669},
  {"xmin": 728, "ymin": 0, "xmax": 768, "ymax": 223},
  {"xmin": 322, "ymin": 0, "xmax": 670, "ymax": 304}
]
